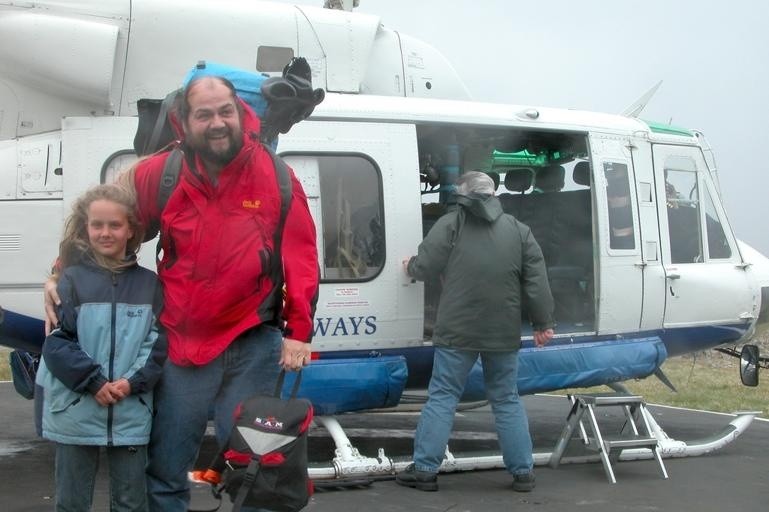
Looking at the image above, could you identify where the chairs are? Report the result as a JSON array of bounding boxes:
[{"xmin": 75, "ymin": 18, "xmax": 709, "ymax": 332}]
[{"xmin": 485, "ymin": 161, "xmax": 594, "ymax": 280}]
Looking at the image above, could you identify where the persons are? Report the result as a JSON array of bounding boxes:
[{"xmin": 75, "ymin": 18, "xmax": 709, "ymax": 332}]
[
  {"xmin": 31, "ymin": 183, "xmax": 170, "ymax": 512},
  {"xmin": 43, "ymin": 74, "xmax": 322, "ymax": 512},
  {"xmin": 395, "ymin": 169, "xmax": 558, "ymax": 493}
]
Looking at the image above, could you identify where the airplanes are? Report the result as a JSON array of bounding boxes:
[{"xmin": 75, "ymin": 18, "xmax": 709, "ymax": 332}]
[{"xmin": 0, "ymin": 0, "xmax": 767, "ymax": 488}]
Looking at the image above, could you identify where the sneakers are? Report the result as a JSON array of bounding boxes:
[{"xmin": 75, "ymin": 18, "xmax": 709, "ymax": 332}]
[
  {"xmin": 397, "ymin": 463, "xmax": 439, "ymax": 491},
  {"xmin": 513, "ymin": 471, "xmax": 536, "ymax": 491}
]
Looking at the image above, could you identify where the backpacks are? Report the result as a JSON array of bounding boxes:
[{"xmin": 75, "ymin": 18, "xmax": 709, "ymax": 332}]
[
  {"xmin": 201, "ymin": 395, "xmax": 314, "ymax": 512},
  {"xmin": 132, "ymin": 63, "xmax": 293, "ymax": 308}
]
